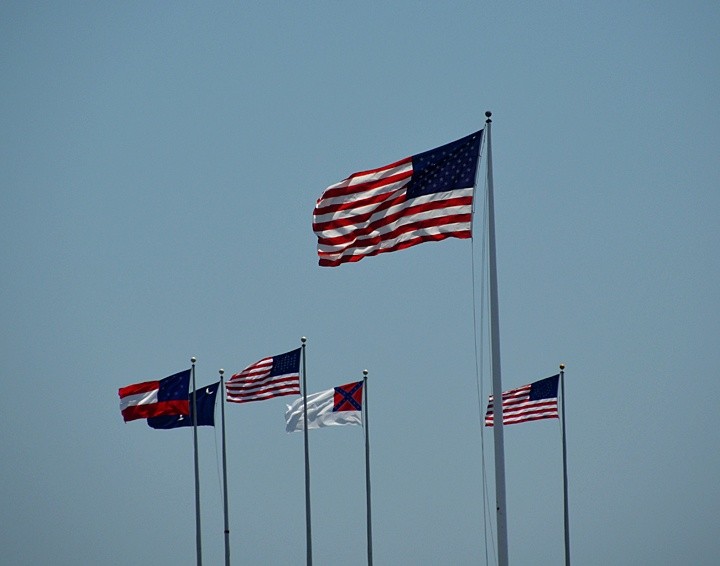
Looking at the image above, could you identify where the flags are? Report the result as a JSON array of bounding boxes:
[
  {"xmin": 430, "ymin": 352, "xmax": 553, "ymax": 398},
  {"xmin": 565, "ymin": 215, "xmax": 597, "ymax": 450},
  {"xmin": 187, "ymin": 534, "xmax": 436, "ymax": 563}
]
[
  {"xmin": 485, "ymin": 375, "xmax": 559, "ymax": 426},
  {"xmin": 118, "ymin": 369, "xmax": 192, "ymax": 424},
  {"xmin": 225, "ymin": 347, "xmax": 300, "ymax": 404},
  {"xmin": 285, "ymin": 380, "xmax": 364, "ymax": 433},
  {"xmin": 313, "ymin": 129, "xmax": 484, "ymax": 267},
  {"xmin": 148, "ymin": 381, "xmax": 216, "ymax": 429}
]
